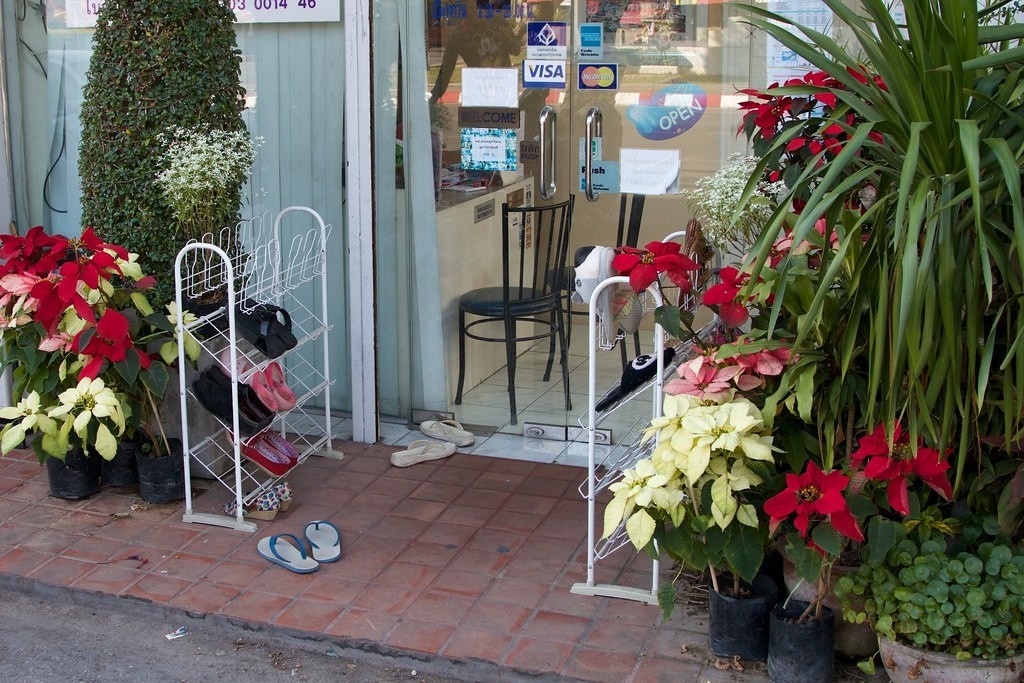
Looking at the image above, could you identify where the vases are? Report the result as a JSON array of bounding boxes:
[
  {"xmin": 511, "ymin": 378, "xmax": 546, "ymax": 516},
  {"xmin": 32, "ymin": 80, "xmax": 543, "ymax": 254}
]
[
  {"xmin": 135, "ymin": 437, "xmax": 188, "ymax": 502},
  {"xmin": 45, "ymin": 452, "xmax": 105, "ymax": 500},
  {"xmin": 103, "ymin": 441, "xmax": 139, "ymax": 488},
  {"xmin": 767, "ymin": 600, "xmax": 835, "ymax": 683},
  {"xmin": 708, "ymin": 575, "xmax": 771, "ymax": 662}
]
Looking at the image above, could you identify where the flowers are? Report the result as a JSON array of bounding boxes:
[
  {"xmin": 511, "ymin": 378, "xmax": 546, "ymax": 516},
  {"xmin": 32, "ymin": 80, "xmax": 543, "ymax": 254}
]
[
  {"xmin": 1, "ymin": 223, "xmax": 202, "ymax": 467},
  {"xmin": 599, "ymin": 0, "xmax": 1024, "ymax": 647}
]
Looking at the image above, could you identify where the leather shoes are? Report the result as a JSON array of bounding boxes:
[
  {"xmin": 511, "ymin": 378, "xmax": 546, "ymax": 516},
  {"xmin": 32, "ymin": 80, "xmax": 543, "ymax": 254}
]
[{"xmin": 194, "ymin": 365, "xmax": 275, "ymax": 436}]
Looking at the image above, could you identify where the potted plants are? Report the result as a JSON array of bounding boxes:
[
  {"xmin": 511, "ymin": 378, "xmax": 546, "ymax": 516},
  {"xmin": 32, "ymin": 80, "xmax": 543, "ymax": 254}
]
[
  {"xmin": 828, "ymin": 511, "xmax": 1024, "ymax": 682},
  {"xmin": 78, "ymin": 0, "xmax": 269, "ymax": 479}
]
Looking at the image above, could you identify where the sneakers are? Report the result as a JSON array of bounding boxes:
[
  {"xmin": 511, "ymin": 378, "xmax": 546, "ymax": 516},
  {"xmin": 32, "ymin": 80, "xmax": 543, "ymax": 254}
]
[
  {"xmin": 573, "ymin": 245, "xmax": 642, "ymax": 343},
  {"xmin": 225, "ymin": 428, "xmax": 299, "ymax": 477}
]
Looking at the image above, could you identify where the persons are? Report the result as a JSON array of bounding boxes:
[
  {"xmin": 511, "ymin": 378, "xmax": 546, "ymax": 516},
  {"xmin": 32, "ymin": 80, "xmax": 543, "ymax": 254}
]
[
  {"xmin": 560, "ymin": 17, "xmax": 626, "ymax": 157},
  {"xmin": 429, "ymin": 1, "xmax": 527, "ymax": 106}
]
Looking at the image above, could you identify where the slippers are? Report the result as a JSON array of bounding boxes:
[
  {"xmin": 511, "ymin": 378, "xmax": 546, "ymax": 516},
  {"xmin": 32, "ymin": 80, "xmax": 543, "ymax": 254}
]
[
  {"xmin": 595, "ymin": 348, "xmax": 675, "ymax": 410},
  {"xmin": 258, "ymin": 520, "xmax": 343, "ymax": 573},
  {"xmin": 390, "ymin": 419, "xmax": 475, "ymax": 467},
  {"xmin": 225, "ymin": 296, "xmax": 298, "ymax": 360},
  {"xmin": 221, "ymin": 348, "xmax": 297, "ymax": 411}
]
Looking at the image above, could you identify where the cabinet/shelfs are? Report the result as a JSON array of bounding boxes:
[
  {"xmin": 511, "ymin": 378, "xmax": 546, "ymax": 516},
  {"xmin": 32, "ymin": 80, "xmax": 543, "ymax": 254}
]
[
  {"xmin": 373, "ymin": 167, "xmax": 536, "ymax": 413},
  {"xmin": 175, "ymin": 206, "xmax": 345, "ymax": 533}
]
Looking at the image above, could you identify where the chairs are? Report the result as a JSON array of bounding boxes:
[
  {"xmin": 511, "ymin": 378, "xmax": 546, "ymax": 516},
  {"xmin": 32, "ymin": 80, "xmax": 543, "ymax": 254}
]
[
  {"xmin": 542, "ymin": 193, "xmax": 646, "ymax": 382},
  {"xmin": 455, "ymin": 195, "xmax": 575, "ymax": 425}
]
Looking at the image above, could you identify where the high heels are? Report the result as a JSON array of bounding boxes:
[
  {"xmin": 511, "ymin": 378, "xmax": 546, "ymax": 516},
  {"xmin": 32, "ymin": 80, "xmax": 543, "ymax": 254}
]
[{"xmin": 223, "ymin": 482, "xmax": 293, "ymax": 520}]
